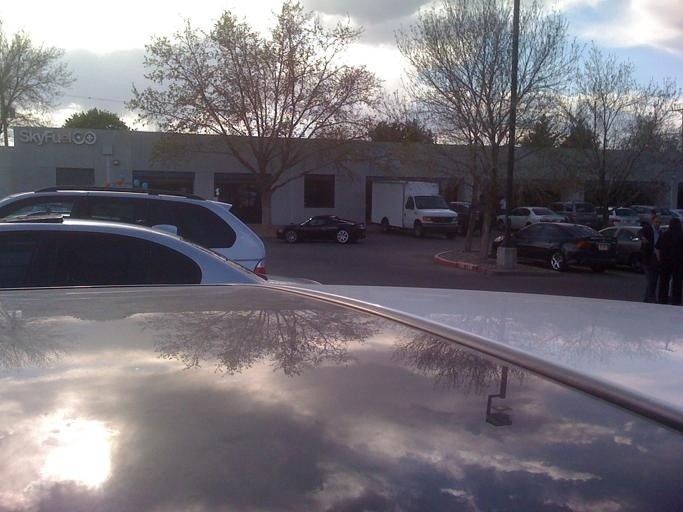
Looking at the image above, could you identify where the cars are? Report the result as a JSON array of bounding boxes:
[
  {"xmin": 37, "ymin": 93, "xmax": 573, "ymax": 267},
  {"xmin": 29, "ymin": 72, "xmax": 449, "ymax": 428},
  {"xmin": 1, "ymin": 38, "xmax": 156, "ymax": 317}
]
[
  {"xmin": 558, "ymin": 198, "xmax": 683, "ymax": 272},
  {"xmin": 0, "ymin": 282, "xmax": 683, "ymax": 512},
  {"xmin": 487, "ymin": 220, "xmax": 620, "ymax": 273},
  {"xmin": 449, "ymin": 199, "xmax": 481, "ymax": 229},
  {"xmin": 495, "ymin": 205, "xmax": 567, "ymax": 231},
  {"xmin": 0, "ymin": 212, "xmax": 322, "ymax": 289}
]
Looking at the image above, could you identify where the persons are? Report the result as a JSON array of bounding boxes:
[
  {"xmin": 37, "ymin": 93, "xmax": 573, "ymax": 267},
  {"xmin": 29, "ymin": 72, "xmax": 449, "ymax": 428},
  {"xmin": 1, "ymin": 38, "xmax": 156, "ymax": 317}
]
[
  {"xmin": 653, "ymin": 217, "xmax": 682, "ymax": 305},
  {"xmin": 636, "ymin": 213, "xmax": 663, "ymax": 304}
]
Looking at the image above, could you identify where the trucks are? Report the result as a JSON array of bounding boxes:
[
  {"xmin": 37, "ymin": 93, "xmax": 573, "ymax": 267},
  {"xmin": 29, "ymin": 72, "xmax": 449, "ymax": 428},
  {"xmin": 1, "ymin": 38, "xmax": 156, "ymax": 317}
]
[{"xmin": 368, "ymin": 178, "xmax": 460, "ymax": 240}]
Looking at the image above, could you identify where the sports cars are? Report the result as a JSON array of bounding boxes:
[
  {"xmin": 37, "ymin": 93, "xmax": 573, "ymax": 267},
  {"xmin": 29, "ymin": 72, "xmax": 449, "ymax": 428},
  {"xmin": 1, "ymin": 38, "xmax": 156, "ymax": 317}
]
[{"xmin": 275, "ymin": 214, "xmax": 368, "ymax": 245}]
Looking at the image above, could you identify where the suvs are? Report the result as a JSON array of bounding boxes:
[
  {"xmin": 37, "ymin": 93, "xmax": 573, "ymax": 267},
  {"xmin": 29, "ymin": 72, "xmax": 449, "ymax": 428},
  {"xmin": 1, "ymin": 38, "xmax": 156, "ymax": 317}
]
[{"xmin": 0, "ymin": 182, "xmax": 269, "ymax": 273}]
